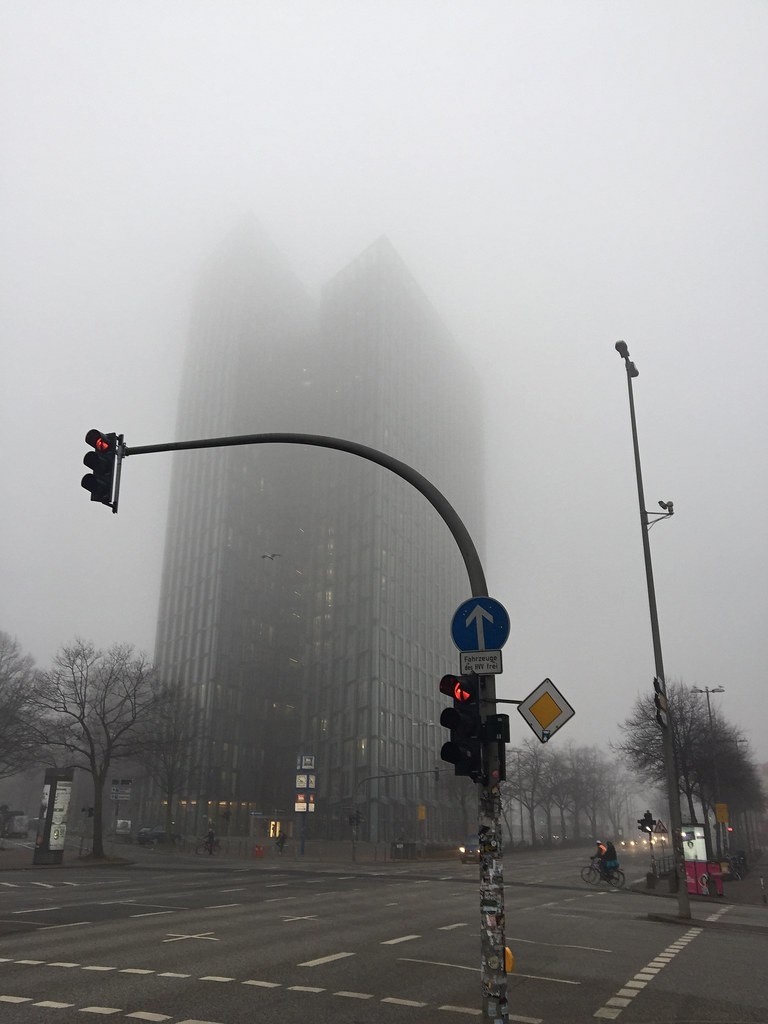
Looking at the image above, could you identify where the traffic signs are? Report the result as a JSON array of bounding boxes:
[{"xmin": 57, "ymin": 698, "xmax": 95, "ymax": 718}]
[{"xmin": 459, "ymin": 649, "xmax": 505, "ymax": 676}]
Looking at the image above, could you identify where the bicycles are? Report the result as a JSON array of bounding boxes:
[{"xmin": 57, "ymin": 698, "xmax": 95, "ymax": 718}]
[
  {"xmin": 196, "ymin": 837, "xmax": 221, "ymax": 856},
  {"xmin": 268, "ymin": 839, "xmax": 291, "ymax": 857},
  {"xmin": 581, "ymin": 856, "xmax": 619, "ymax": 884},
  {"xmin": 588, "ymin": 856, "xmax": 625, "ymax": 889}
]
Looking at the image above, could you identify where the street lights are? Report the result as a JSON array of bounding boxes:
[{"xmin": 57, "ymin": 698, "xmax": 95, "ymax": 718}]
[
  {"xmin": 688, "ymin": 685, "xmax": 733, "ymax": 880},
  {"xmin": 611, "ymin": 337, "xmax": 692, "ymax": 918}
]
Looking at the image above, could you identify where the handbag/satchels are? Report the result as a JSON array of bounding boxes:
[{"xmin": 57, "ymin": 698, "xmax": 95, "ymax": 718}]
[{"xmin": 605, "ymin": 860, "xmax": 619, "ymax": 868}]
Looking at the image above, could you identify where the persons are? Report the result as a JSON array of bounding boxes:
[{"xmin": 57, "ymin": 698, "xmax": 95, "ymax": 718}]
[
  {"xmin": 590, "ymin": 840, "xmax": 606, "ymax": 860},
  {"xmin": 203, "ymin": 829, "xmax": 215, "ymax": 855},
  {"xmin": 276, "ymin": 830, "xmax": 285, "ymax": 854},
  {"xmin": 600, "ymin": 841, "xmax": 617, "ymax": 878}
]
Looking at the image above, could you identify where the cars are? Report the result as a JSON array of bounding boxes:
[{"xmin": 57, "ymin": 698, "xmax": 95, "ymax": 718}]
[{"xmin": 459, "ymin": 844, "xmax": 480, "ymax": 864}]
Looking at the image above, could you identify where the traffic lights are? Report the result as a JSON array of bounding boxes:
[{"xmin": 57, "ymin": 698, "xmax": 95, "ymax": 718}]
[
  {"xmin": 439, "ymin": 671, "xmax": 483, "ymax": 777},
  {"xmin": 637, "ymin": 818, "xmax": 645, "ymax": 832},
  {"xmin": 82, "ymin": 428, "xmax": 117, "ymax": 504},
  {"xmin": 727, "ymin": 826, "xmax": 733, "ymax": 834}
]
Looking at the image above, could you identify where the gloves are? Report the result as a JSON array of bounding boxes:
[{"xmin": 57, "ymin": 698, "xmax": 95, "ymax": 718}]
[{"xmin": 591, "ymin": 856, "xmax": 594, "ymax": 859}]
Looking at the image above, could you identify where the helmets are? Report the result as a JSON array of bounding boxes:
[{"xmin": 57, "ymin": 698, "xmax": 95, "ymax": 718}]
[{"xmin": 596, "ymin": 840, "xmax": 601, "ymax": 843}]
